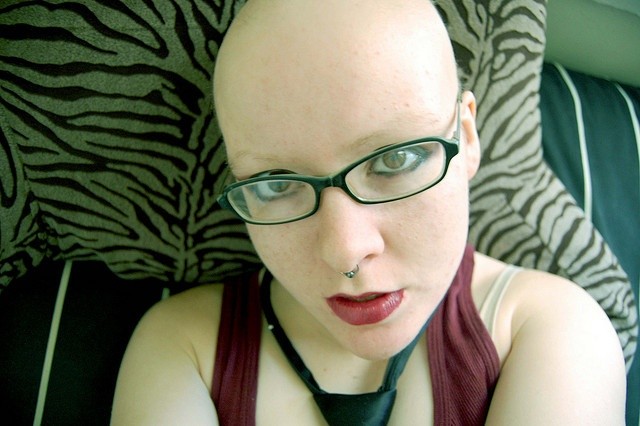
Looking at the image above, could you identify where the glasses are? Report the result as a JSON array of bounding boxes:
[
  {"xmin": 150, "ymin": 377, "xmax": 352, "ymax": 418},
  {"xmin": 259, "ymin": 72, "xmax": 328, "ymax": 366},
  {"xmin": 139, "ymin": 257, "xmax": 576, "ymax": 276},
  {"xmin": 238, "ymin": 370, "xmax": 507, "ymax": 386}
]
[{"xmin": 218, "ymin": 93, "xmax": 460, "ymax": 225}]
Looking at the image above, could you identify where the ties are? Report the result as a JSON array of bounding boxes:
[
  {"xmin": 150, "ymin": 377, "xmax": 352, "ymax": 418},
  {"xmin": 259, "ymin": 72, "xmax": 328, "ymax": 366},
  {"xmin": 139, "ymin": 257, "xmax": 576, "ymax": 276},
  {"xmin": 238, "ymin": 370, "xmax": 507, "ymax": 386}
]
[{"xmin": 260, "ymin": 270, "xmax": 433, "ymax": 426}]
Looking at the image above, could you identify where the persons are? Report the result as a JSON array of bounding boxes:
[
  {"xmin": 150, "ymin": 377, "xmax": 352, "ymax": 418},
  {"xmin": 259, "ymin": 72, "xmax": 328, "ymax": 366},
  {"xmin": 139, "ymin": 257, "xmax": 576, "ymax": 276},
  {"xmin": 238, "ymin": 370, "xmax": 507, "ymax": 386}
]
[{"xmin": 109, "ymin": 1, "xmax": 627, "ymax": 426}]
